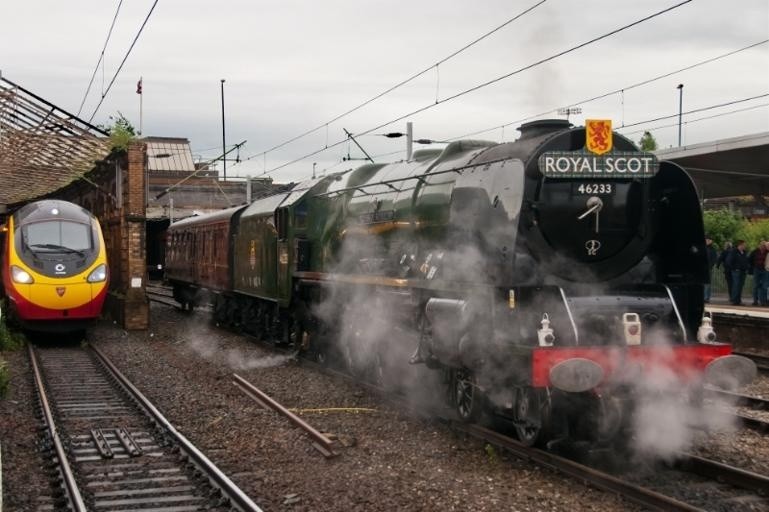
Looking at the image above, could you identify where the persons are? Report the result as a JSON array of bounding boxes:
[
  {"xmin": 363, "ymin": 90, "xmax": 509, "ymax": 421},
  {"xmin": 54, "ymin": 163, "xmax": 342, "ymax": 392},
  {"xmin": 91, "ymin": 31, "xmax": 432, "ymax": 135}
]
[{"xmin": 704, "ymin": 235, "xmax": 769, "ymax": 306}]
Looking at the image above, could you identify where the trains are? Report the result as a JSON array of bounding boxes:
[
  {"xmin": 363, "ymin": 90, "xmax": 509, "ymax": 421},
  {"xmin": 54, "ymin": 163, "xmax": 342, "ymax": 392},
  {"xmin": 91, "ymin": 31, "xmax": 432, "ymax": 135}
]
[
  {"xmin": 162, "ymin": 119, "xmax": 734, "ymax": 461},
  {"xmin": 0, "ymin": 198, "xmax": 111, "ymax": 334}
]
